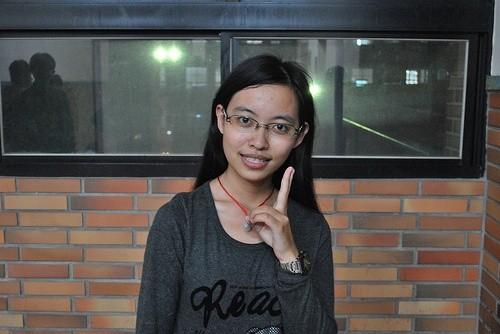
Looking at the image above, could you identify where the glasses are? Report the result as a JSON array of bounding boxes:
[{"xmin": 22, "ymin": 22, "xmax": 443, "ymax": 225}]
[{"xmin": 218, "ymin": 103, "xmax": 304, "ymax": 139}]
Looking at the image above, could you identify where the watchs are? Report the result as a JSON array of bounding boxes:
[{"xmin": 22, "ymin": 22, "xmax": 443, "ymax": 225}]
[{"xmin": 277, "ymin": 251, "xmax": 311, "ymax": 276}]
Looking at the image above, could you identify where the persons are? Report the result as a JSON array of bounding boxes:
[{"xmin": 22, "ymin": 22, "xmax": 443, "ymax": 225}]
[
  {"xmin": 26, "ymin": 54, "xmax": 76, "ymax": 152},
  {"xmin": 135, "ymin": 54, "xmax": 337, "ymax": 333},
  {"xmin": 2, "ymin": 61, "xmax": 61, "ymax": 154},
  {"xmin": 90, "ymin": 57, "xmax": 164, "ymax": 154}
]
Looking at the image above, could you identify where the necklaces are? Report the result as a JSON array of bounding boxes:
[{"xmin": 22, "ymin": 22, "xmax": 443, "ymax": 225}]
[{"xmin": 217, "ymin": 176, "xmax": 275, "ymax": 230}]
